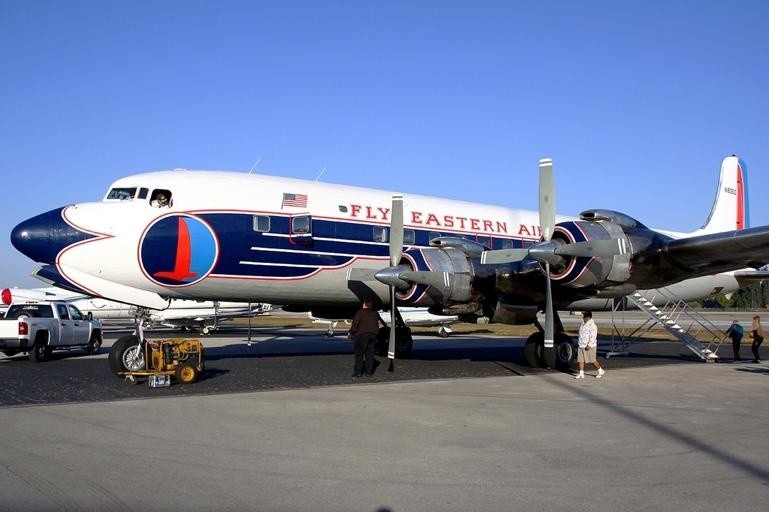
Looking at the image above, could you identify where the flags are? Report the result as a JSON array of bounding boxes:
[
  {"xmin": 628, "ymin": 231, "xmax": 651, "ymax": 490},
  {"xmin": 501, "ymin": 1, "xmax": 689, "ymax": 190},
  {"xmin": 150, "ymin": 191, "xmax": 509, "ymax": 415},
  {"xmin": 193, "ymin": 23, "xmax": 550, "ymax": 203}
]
[{"xmin": 282, "ymin": 193, "xmax": 307, "ymax": 208}]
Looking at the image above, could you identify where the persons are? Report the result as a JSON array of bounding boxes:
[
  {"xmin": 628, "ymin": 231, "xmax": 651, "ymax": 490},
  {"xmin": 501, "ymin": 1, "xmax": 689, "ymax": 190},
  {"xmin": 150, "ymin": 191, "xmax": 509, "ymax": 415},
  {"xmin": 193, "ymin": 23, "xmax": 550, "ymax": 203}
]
[
  {"xmin": 748, "ymin": 316, "xmax": 765, "ymax": 363},
  {"xmin": 151, "ymin": 195, "xmax": 169, "ymax": 208},
  {"xmin": 124, "ymin": 191, "xmax": 141, "ymax": 199},
  {"xmin": 573, "ymin": 310, "xmax": 605, "ymax": 379},
  {"xmin": 725, "ymin": 319, "xmax": 744, "ymax": 361},
  {"xmin": 348, "ymin": 296, "xmax": 379, "ymax": 377}
]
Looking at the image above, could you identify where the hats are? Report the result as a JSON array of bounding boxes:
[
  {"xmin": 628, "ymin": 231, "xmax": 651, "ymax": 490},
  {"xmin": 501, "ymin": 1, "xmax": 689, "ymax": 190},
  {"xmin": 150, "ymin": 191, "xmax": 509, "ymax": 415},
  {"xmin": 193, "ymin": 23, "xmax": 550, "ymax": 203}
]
[{"xmin": 582, "ymin": 311, "xmax": 592, "ymax": 316}]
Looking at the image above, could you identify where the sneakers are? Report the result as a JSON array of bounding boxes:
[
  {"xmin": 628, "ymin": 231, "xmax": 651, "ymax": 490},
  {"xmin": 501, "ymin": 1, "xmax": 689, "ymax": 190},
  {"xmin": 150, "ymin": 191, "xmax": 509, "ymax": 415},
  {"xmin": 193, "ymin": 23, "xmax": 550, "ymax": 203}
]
[
  {"xmin": 574, "ymin": 373, "xmax": 584, "ymax": 379},
  {"xmin": 351, "ymin": 373, "xmax": 370, "ymax": 377},
  {"xmin": 595, "ymin": 369, "xmax": 605, "ymax": 379}
]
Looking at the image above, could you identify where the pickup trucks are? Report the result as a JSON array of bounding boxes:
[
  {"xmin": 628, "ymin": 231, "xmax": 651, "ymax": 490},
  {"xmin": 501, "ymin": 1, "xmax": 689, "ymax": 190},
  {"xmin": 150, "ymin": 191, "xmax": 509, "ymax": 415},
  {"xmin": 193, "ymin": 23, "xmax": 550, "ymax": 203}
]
[{"xmin": 1, "ymin": 302, "xmax": 103, "ymax": 362}]
